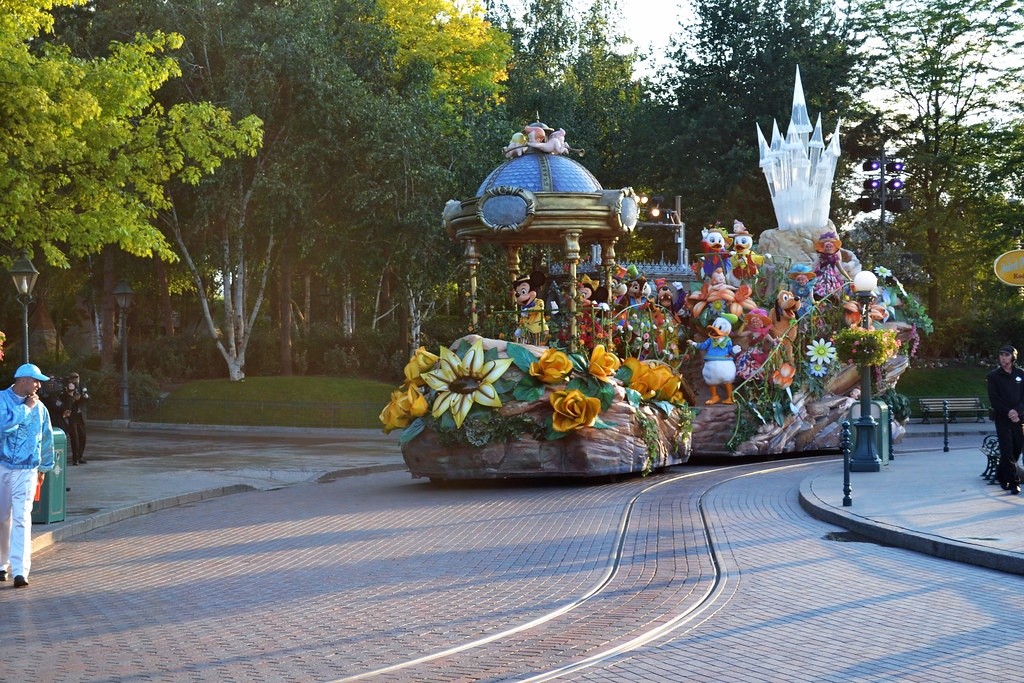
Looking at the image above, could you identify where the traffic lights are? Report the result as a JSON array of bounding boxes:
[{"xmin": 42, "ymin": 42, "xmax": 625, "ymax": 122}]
[{"xmin": 862, "ymin": 159, "xmax": 904, "ymax": 190}]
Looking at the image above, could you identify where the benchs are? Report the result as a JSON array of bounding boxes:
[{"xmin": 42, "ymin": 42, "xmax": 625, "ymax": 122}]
[
  {"xmin": 979, "ymin": 435, "xmax": 1002, "ymax": 485},
  {"xmin": 918, "ymin": 397, "xmax": 994, "ymax": 424}
]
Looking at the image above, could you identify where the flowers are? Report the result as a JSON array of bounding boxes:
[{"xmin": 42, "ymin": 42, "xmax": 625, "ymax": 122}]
[
  {"xmin": 377, "ymin": 338, "xmax": 687, "ymax": 442},
  {"xmin": 873, "ymin": 266, "xmax": 893, "ymax": 278},
  {"xmin": 806, "ymin": 338, "xmax": 836, "ymax": 377},
  {"xmin": 830, "ymin": 325, "xmax": 902, "ymax": 367}
]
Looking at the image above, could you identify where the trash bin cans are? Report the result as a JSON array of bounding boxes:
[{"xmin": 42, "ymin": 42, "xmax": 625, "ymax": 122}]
[
  {"xmin": 31, "ymin": 426, "xmax": 68, "ymax": 526},
  {"xmin": 848, "ymin": 400, "xmax": 890, "ymax": 467}
]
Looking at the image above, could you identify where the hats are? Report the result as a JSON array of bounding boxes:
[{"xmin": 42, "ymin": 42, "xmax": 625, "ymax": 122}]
[{"xmin": 13, "ymin": 363, "xmax": 51, "ymax": 381}]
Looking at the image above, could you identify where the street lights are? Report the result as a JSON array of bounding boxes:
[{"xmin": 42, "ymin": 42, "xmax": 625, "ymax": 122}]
[
  {"xmin": 848, "ymin": 270, "xmax": 882, "ymax": 472},
  {"xmin": 10, "ymin": 248, "xmax": 40, "ymax": 364},
  {"xmin": 112, "ymin": 281, "xmax": 135, "ymax": 420}
]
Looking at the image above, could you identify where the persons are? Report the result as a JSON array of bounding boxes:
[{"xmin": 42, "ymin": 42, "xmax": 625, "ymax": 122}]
[
  {"xmin": 0, "ymin": 363, "xmax": 55, "ymax": 588},
  {"xmin": 786, "ymin": 263, "xmax": 818, "ymax": 317},
  {"xmin": 57, "ymin": 372, "xmax": 90, "ymax": 466},
  {"xmin": 838, "ymin": 388, "xmax": 862, "ymax": 411},
  {"xmin": 987, "ymin": 345, "xmax": 1024, "ymax": 494},
  {"xmin": 731, "ymin": 308, "xmax": 780, "ymax": 383},
  {"xmin": 813, "ymin": 231, "xmax": 852, "ymax": 301}
]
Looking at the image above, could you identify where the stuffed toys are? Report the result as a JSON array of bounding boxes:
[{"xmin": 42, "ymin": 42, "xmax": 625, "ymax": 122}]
[
  {"xmin": 699, "ymin": 219, "xmax": 772, "ymax": 288},
  {"xmin": 557, "ymin": 264, "xmax": 687, "ymax": 368},
  {"xmin": 514, "ymin": 271, "xmax": 549, "ymax": 345},
  {"xmin": 686, "ymin": 313, "xmax": 741, "ymax": 404},
  {"xmin": 767, "ymin": 289, "xmax": 802, "ymax": 365}
]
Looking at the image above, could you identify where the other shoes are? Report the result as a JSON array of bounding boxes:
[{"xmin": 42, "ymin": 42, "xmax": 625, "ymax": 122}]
[
  {"xmin": 1001, "ymin": 481, "xmax": 1010, "ymax": 489},
  {"xmin": 1012, "ymin": 486, "xmax": 1021, "ymax": 494},
  {"xmin": 14, "ymin": 576, "xmax": 29, "ymax": 588},
  {"xmin": 73, "ymin": 460, "xmax": 79, "ymax": 465},
  {"xmin": 79, "ymin": 458, "xmax": 87, "ymax": 464},
  {"xmin": 0, "ymin": 571, "xmax": 8, "ymax": 581}
]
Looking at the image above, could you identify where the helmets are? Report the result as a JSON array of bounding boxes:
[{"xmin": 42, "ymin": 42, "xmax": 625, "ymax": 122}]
[{"xmin": 997, "ymin": 345, "xmax": 1018, "ymax": 360}]
